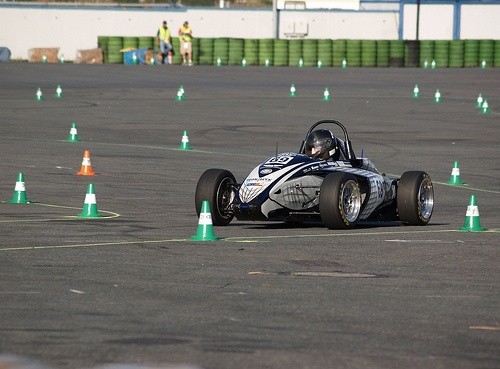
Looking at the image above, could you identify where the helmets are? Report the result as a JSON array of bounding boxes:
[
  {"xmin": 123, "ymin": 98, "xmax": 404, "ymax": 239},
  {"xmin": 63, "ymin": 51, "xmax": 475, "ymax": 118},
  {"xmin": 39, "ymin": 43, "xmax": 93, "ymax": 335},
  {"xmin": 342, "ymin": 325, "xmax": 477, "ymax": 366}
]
[{"xmin": 304, "ymin": 129, "xmax": 338, "ymax": 160}]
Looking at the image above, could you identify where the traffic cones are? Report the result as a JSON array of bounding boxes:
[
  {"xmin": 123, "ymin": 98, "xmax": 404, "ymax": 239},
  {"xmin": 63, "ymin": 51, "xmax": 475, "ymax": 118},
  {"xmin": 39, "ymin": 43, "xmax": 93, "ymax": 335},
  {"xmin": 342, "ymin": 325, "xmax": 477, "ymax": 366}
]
[
  {"xmin": 178, "ymin": 130, "xmax": 193, "ymax": 150},
  {"xmin": 216, "ymin": 57, "xmax": 347, "ymax": 68},
  {"xmin": 176, "ymin": 84, "xmax": 186, "ymax": 102},
  {"xmin": 321, "ymin": 87, "xmax": 330, "ymax": 102},
  {"xmin": 191, "ymin": 200, "xmax": 220, "ymax": 241},
  {"xmin": 447, "ymin": 160, "xmax": 465, "ymax": 186},
  {"xmin": 475, "ymin": 90, "xmax": 484, "ymax": 110},
  {"xmin": 288, "ymin": 83, "xmax": 297, "ymax": 98},
  {"xmin": 78, "ymin": 184, "xmax": 103, "ymax": 218},
  {"xmin": 481, "ymin": 61, "xmax": 487, "ymax": 71},
  {"xmin": 413, "ymin": 84, "xmax": 419, "ymax": 98},
  {"xmin": 458, "ymin": 193, "xmax": 488, "ymax": 232},
  {"xmin": 479, "ymin": 97, "xmax": 491, "ymax": 114},
  {"xmin": 76, "ymin": 151, "xmax": 96, "ymax": 176},
  {"xmin": 431, "ymin": 60, "xmax": 436, "ymax": 71},
  {"xmin": 424, "ymin": 60, "xmax": 429, "ymax": 71},
  {"xmin": 434, "ymin": 88, "xmax": 441, "ymax": 104},
  {"xmin": 7, "ymin": 172, "xmax": 33, "ymax": 205},
  {"xmin": 35, "ymin": 54, "xmax": 80, "ymax": 143}
]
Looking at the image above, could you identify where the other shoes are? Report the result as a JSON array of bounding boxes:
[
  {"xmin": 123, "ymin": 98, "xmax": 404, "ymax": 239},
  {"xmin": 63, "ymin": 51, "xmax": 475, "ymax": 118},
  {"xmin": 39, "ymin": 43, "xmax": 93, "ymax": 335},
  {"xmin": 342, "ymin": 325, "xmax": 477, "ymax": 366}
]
[{"xmin": 188, "ymin": 63, "xmax": 192, "ymax": 67}]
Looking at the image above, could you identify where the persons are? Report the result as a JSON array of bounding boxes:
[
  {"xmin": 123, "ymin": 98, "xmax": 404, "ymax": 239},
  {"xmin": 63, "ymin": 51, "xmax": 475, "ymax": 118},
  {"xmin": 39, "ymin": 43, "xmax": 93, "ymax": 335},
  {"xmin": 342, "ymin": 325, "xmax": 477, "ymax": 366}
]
[
  {"xmin": 156, "ymin": 21, "xmax": 175, "ymax": 63},
  {"xmin": 305, "ymin": 129, "xmax": 337, "ymax": 160},
  {"xmin": 179, "ymin": 21, "xmax": 193, "ymax": 66}
]
